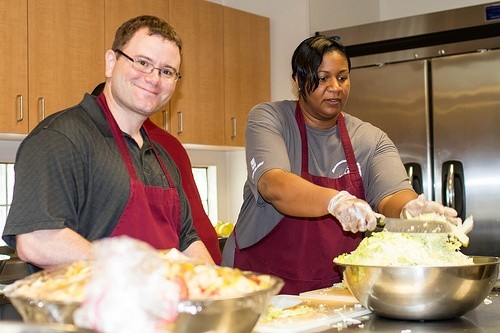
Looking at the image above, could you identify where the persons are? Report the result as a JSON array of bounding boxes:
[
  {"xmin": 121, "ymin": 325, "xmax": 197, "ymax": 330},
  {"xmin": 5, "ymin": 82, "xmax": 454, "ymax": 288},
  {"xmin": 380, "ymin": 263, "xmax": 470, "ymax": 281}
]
[
  {"xmin": 90, "ymin": 82, "xmax": 222, "ymax": 266},
  {"xmin": 2, "ymin": 15, "xmax": 219, "ymax": 277},
  {"xmin": 219, "ymin": 34, "xmax": 462, "ymax": 296}
]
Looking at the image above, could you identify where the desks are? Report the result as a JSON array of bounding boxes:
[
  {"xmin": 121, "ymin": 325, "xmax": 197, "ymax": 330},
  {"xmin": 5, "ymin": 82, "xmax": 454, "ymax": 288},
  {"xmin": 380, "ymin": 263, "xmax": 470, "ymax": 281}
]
[{"xmin": 316, "ymin": 289, "xmax": 500, "ymax": 333}]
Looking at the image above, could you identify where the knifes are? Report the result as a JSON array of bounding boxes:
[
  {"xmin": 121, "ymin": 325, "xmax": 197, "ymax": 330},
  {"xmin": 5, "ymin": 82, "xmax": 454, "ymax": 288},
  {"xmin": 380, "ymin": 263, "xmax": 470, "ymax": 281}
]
[{"xmin": 366, "ymin": 216, "xmax": 455, "ymax": 234}]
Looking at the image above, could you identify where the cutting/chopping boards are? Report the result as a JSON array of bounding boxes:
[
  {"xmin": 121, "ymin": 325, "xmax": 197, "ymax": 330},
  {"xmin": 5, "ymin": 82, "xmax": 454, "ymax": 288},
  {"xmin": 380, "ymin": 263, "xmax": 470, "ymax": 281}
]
[
  {"xmin": 299, "ymin": 282, "xmax": 356, "ymax": 300},
  {"xmin": 250, "ymin": 293, "xmax": 374, "ymax": 332}
]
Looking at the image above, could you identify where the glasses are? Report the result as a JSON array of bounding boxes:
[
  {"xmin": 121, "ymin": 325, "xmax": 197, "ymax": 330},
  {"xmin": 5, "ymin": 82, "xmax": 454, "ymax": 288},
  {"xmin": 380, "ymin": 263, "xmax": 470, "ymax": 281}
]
[{"xmin": 114, "ymin": 48, "xmax": 182, "ymax": 82}]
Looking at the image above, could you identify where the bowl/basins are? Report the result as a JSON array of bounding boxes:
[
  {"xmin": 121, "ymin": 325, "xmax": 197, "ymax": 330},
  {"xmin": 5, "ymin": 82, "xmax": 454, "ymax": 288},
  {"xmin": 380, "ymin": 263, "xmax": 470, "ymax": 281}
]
[
  {"xmin": 333, "ymin": 254, "xmax": 500, "ymax": 320},
  {"xmin": 0, "ymin": 270, "xmax": 285, "ymax": 333}
]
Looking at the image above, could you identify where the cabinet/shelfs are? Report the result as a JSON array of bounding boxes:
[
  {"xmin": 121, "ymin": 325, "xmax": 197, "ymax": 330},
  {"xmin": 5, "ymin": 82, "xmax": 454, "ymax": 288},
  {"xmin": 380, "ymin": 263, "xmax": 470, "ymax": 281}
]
[{"xmin": 0, "ymin": 0, "xmax": 271, "ymax": 147}]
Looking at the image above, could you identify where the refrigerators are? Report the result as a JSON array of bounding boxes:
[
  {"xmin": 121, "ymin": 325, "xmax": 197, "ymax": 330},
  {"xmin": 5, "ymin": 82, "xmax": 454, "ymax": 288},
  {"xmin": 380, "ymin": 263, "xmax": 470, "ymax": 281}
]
[{"xmin": 316, "ymin": 1, "xmax": 500, "ymax": 290}]
